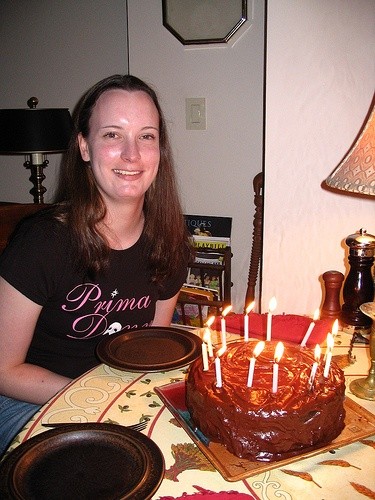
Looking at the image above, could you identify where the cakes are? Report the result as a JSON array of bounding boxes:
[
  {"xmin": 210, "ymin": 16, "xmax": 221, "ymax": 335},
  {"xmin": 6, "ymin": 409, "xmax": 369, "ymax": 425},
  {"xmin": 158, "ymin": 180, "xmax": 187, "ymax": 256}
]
[{"xmin": 185, "ymin": 339, "xmax": 346, "ymax": 463}]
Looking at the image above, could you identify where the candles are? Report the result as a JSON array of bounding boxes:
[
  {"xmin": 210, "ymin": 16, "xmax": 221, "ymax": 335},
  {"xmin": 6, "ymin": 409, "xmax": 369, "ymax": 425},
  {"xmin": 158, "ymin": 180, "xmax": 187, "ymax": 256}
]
[
  {"xmin": 272, "ymin": 342, "xmax": 284, "ymax": 393},
  {"xmin": 201, "ymin": 315, "xmax": 215, "ymax": 371},
  {"xmin": 244, "ymin": 301, "xmax": 255, "ymax": 342},
  {"xmin": 301, "ymin": 309, "xmax": 319, "ymax": 347},
  {"xmin": 307, "ymin": 344, "xmax": 321, "ymax": 390},
  {"xmin": 214, "ymin": 346, "xmax": 227, "ymax": 388},
  {"xmin": 246, "ymin": 341, "xmax": 266, "ymax": 387},
  {"xmin": 220, "ymin": 305, "xmax": 233, "ymax": 347},
  {"xmin": 266, "ymin": 297, "xmax": 277, "ymax": 342},
  {"xmin": 323, "ymin": 319, "xmax": 338, "ymax": 377}
]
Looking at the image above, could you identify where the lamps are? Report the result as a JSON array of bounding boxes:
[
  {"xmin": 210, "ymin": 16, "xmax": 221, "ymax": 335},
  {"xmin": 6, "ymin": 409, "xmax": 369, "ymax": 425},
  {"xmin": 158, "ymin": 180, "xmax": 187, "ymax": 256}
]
[
  {"xmin": 319, "ymin": 87, "xmax": 375, "ymax": 401},
  {"xmin": 0, "ymin": 97, "xmax": 72, "ymax": 205}
]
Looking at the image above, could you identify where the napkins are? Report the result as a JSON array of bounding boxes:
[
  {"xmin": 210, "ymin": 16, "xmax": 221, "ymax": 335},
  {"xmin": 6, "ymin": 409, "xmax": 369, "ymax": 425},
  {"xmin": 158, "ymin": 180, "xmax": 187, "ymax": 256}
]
[{"xmin": 210, "ymin": 312, "xmax": 334, "ymax": 348}]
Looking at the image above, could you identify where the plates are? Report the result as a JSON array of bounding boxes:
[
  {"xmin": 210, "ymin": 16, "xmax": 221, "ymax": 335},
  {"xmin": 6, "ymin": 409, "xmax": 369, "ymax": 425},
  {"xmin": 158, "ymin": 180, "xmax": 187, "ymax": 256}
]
[
  {"xmin": 0, "ymin": 422, "xmax": 165, "ymax": 500},
  {"xmin": 96, "ymin": 326, "xmax": 203, "ymax": 373}
]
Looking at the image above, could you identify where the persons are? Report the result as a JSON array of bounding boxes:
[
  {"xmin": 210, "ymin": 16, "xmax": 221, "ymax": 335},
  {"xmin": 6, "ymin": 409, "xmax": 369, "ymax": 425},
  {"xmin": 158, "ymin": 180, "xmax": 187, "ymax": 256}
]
[{"xmin": 0, "ymin": 76, "xmax": 195, "ymax": 463}]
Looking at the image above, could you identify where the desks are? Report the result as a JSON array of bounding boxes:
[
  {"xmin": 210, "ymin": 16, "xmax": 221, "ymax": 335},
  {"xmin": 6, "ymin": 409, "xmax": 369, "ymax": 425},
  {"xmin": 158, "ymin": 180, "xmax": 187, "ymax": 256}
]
[{"xmin": 0, "ymin": 318, "xmax": 375, "ymax": 499}]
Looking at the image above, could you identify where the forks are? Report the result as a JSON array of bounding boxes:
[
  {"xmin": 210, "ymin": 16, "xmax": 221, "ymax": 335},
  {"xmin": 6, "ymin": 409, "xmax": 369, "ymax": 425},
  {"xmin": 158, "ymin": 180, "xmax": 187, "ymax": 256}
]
[{"xmin": 41, "ymin": 422, "xmax": 147, "ymax": 431}]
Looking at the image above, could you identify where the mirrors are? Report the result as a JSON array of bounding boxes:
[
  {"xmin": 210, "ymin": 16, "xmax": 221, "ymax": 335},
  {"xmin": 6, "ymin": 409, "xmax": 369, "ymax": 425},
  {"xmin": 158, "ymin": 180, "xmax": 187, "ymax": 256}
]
[{"xmin": 161, "ymin": 0, "xmax": 248, "ymax": 46}]
